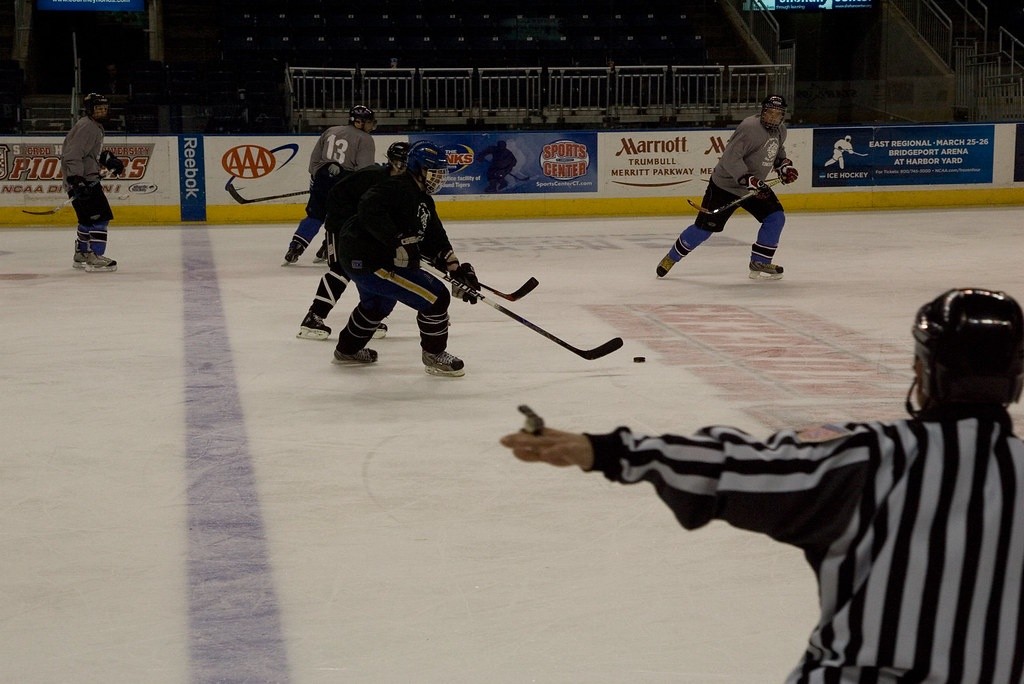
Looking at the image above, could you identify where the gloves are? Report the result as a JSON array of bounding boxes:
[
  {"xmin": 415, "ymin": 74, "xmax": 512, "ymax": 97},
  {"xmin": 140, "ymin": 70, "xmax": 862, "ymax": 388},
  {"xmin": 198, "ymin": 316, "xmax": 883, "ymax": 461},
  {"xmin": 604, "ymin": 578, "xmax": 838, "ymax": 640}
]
[
  {"xmin": 66, "ymin": 175, "xmax": 92, "ymax": 201},
  {"xmin": 450, "ymin": 263, "xmax": 481, "ymax": 304},
  {"xmin": 99, "ymin": 150, "xmax": 124, "ymax": 176},
  {"xmin": 387, "ymin": 232, "xmax": 421, "ymax": 271},
  {"xmin": 774, "ymin": 156, "xmax": 799, "ymax": 185},
  {"xmin": 738, "ymin": 174, "xmax": 771, "ymax": 201},
  {"xmin": 309, "ymin": 174, "xmax": 315, "ymax": 194},
  {"xmin": 428, "ymin": 254, "xmax": 447, "ymax": 273}
]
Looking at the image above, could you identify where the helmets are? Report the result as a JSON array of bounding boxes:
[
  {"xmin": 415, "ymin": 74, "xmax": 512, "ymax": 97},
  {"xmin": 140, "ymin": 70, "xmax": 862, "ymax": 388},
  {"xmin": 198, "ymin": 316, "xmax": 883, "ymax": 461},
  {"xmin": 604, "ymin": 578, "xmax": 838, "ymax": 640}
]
[
  {"xmin": 913, "ymin": 287, "xmax": 1024, "ymax": 406},
  {"xmin": 83, "ymin": 93, "xmax": 110, "ymax": 124},
  {"xmin": 406, "ymin": 140, "xmax": 449, "ymax": 195},
  {"xmin": 386, "ymin": 140, "xmax": 413, "ymax": 174},
  {"xmin": 349, "ymin": 106, "xmax": 374, "ymax": 133},
  {"xmin": 760, "ymin": 95, "xmax": 787, "ymax": 131}
]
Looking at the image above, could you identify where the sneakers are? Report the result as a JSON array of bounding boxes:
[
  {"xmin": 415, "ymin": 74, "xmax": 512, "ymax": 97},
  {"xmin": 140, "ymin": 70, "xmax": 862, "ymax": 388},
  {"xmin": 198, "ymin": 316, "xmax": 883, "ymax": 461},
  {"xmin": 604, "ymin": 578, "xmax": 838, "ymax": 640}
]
[
  {"xmin": 370, "ymin": 323, "xmax": 388, "ymax": 339},
  {"xmin": 295, "ymin": 303, "xmax": 331, "ymax": 340},
  {"xmin": 656, "ymin": 254, "xmax": 676, "ymax": 278},
  {"xmin": 422, "ymin": 347, "xmax": 466, "ymax": 377},
  {"xmin": 281, "ymin": 243, "xmax": 305, "ymax": 266},
  {"xmin": 73, "ymin": 239, "xmax": 93, "ymax": 268},
  {"xmin": 748, "ymin": 258, "xmax": 783, "ymax": 280},
  {"xmin": 85, "ymin": 251, "xmax": 117, "ymax": 272},
  {"xmin": 332, "ymin": 331, "xmax": 377, "ymax": 366},
  {"xmin": 313, "ymin": 240, "xmax": 328, "ymax": 264}
]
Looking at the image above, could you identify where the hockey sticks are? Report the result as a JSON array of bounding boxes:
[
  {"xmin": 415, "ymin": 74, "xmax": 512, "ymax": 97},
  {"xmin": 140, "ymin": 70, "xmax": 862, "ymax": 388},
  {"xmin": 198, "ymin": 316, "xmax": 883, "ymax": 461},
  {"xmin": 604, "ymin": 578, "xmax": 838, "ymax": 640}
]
[
  {"xmin": 418, "ymin": 260, "xmax": 623, "ymax": 360},
  {"xmin": 416, "ymin": 251, "xmax": 539, "ymax": 302},
  {"xmin": 687, "ymin": 177, "xmax": 787, "ymax": 216},
  {"xmin": 224, "ymin": 181, "xmax": 309, "ymax": 205},
  {"xmin": 843, "ymin": 148, "xmax": 869, "ymax": 156},
  {"xmin": 481, "ymin": 157, "xmax": 530, "ymax": 181},
  {"xmin": 21, "ymin": 169, "xmax": 116, "ymax": 215}
]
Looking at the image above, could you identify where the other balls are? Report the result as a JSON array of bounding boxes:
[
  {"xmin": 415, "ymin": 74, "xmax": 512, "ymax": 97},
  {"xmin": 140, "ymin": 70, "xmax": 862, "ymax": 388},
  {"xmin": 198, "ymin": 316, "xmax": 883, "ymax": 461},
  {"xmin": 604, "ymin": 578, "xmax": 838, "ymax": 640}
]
[{"xmin": 634, "ymin": 356, "xmax": 646, "ymax": 363}]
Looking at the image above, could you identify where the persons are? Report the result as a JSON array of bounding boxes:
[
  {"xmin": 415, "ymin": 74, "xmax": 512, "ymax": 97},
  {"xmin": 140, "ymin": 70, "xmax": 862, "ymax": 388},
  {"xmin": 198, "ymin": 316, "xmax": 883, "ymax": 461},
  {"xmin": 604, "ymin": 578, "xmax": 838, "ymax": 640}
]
[
  {"xmin": 61, "ymin": 93, "xmax": 125, "ymax": 272},
  {"xmin": 331, "ymin": 140, "xmax": 480, "ymax": 376},
  {"xmin": 296, "ymin": 140, "xmax": 415, "ymax": 340},
  {"xmin": 656, "ymin": 94, "xmax": 799, "ymax": 281},
  {"xmin": 500, "ymin": 287, "xmax": 1024, "ymax": 684},
  {"xmin": 281, "ymin": 105, "xmax": 376, "ymax": 265}
]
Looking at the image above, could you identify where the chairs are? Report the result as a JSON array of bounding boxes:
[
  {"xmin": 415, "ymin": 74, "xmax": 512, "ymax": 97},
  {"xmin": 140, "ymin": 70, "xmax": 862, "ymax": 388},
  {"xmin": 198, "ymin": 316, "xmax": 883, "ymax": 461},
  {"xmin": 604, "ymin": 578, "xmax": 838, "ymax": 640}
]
[{"xmin": 130, "ymin": 0, "xmax": 778, "ymax": 130}]
